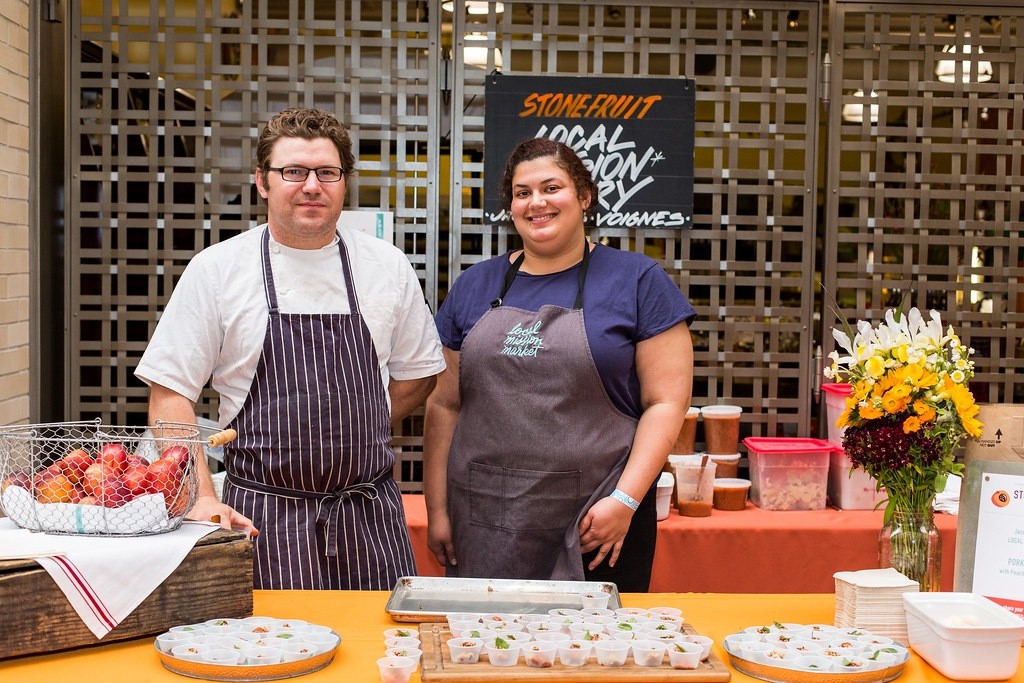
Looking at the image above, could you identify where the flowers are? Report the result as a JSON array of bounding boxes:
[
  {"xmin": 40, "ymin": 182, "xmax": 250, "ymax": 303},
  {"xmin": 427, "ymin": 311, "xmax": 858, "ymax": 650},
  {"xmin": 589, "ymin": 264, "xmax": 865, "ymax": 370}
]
[{"xmin": 818, "ymin": 280, "xmax": 984, "ymax": 593}]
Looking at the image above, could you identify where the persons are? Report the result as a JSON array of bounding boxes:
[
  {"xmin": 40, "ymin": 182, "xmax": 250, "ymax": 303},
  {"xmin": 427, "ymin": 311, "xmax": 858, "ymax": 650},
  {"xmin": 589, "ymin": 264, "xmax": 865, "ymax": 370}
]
[
  {"xmin": 420, "ymin": 137, "xmax": 699, "ymax": 592},
  {"xmin": 134, "ymin": 106, "xmax": 447, "ymax": 590}
]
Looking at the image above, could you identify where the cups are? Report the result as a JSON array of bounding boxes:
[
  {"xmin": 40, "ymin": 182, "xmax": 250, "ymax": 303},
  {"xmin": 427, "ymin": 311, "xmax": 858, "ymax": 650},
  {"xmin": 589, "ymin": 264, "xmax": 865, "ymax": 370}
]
[
  {"xmin": 701, "ymin": 405, "xmax": 742, "ymax": 454},
  {"xmin": 668, "ymin": 407, "xmax": 702, "ymax": 455},
  {"xmin": 664, "ymin": 451, "xmax": 752, "ymax": 517}
]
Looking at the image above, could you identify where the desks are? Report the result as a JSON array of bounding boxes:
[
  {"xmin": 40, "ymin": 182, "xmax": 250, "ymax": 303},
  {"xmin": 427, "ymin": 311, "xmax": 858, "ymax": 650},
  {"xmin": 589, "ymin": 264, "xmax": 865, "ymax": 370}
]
[
  {"xmin": 0, "ymin": 588, "xmax": 1024, "ymax": 683},
  {"xmin": 400, "ymin": 494, "xmax": 958, "ymax": 594}
]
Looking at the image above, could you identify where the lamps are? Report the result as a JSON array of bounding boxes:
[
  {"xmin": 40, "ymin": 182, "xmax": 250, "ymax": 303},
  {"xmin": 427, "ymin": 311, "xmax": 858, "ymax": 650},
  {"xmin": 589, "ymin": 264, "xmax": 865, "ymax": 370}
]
[
  {"xmin": 936, "ymin": 17, "xmax": 992, "ymax": 83},
  {"xmin": 440, "ymin": 0, "xmax": 504, "ymax": 15},
  {"xmin": 447, "ymin": 21, "xmax": 503, "ymax": 70},
  {"xmin": 843, "ymin": 89, "xmax": 879, "ymax": 123}
]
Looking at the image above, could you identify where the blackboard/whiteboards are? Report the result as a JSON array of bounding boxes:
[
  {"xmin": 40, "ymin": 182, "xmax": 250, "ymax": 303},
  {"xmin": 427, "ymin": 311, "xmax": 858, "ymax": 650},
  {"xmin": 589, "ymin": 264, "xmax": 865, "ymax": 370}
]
[{"xmin": 483, "ymin": 73, "xmax": 696, "ymax": 231}]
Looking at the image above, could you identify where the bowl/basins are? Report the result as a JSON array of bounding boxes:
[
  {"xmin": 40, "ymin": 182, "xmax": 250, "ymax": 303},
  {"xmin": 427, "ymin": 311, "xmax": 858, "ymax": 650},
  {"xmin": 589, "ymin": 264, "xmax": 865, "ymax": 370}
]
[
  {"xmin": 377, "ymin": 658, "xmax": 415, "ymax": 683},
  {"xmin": 581, "ymin": 592, "xmax": 611, "ymax": 609},
  {"xmin": 677, "ymin": 635, "xmax": 713, "ymax": 661},
  {"xmin": 385, "ymin": 647, "xmax": 422, "ymax": 673},
  {"xmin": 384, "ymin": 629, "xmax": 420, "ymax": 639},
  {"xmin": 384, "ymin": 637, "xmax": 421, "ymax": 649},
  {"xmin": 666, "ymin": 642, "xmax": 704, "ymax": 669},
  {"xmin": 447, "ymin": 608, "xmax": 684, "ymax": 666},
  {"xmin": 156, "ymin": 617, "xmax": 340, "ymax": 665},
  {"xmin": 726, "ymin": 623, "xmax": 908, "ymax": 672}
]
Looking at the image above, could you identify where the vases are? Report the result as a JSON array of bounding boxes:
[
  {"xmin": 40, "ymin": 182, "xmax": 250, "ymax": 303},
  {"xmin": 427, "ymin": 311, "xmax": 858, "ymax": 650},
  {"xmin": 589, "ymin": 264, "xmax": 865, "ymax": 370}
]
[{"xmin": 877, "ymin": 507, "xmax": 942, "ymax": 593}]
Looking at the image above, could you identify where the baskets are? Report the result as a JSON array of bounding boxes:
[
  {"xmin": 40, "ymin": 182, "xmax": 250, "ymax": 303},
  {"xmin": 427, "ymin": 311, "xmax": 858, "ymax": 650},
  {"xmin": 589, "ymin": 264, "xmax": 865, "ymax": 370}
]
[{"xmin": 1, "ymin": 418, "xmax": 200, "ymax": 536}]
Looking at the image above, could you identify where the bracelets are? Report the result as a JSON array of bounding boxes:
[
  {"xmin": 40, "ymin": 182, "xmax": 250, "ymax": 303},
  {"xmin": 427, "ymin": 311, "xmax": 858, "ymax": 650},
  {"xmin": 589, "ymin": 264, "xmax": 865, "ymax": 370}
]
[{"xmin": 611, "ymin": 490, "xmax": 640, "ymax": 511}]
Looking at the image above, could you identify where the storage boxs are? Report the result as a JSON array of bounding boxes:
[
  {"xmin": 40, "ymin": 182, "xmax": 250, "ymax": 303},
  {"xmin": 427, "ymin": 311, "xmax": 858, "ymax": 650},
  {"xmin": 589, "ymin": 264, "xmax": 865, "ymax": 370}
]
[
  {"xmin": 821, "ymin": 381, "xmax": 889, "ymax": 510},
  {"xmin": 742, "ymin": 437, "xmax": 835, "ymax": 509},
  {"xmin": 964, "ymin": 402, "xmax": 1024, "ymax": 463}
]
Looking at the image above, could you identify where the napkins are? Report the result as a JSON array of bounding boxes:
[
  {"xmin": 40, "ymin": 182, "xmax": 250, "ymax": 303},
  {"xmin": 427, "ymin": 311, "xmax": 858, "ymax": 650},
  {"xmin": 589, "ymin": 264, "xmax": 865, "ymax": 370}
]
[{"xmin": 831, "ymin": 565, "xmax": 920, "ymax": 648}]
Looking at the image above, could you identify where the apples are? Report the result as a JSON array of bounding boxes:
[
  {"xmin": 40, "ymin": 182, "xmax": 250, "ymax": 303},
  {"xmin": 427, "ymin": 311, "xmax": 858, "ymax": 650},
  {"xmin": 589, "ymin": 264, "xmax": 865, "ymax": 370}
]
[{"xmin": 0, "ymin": 444, "xmax": 196, "ymax": 519}]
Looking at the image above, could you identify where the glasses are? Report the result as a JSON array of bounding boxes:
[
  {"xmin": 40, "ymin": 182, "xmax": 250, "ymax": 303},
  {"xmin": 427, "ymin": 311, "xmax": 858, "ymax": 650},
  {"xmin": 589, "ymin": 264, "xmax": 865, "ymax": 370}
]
[{"xmin": 261, "ymin": 163, "xmax": 347, "ymax": 184}]
